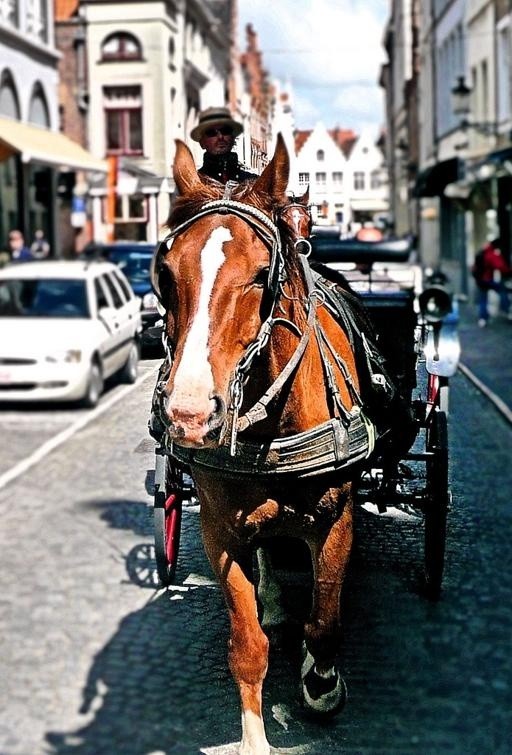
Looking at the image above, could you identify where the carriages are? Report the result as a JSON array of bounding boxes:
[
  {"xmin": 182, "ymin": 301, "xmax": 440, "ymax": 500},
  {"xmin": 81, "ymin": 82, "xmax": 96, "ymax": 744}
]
[{"xmin": 148, "ymin": 131, "xmax": 462, "ymax": 755}]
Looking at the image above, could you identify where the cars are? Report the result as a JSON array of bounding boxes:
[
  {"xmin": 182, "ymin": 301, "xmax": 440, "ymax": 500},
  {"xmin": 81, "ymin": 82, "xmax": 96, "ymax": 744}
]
[{"xmin": 0, "ymin": 243, "xmax": 166, "ymax": 407}]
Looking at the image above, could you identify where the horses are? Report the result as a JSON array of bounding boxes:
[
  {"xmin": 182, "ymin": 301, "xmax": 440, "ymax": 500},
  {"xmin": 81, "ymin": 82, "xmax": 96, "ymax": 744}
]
[{"xmin": 156, "ymin": 131, "xmax": 384, "ymax": 755}]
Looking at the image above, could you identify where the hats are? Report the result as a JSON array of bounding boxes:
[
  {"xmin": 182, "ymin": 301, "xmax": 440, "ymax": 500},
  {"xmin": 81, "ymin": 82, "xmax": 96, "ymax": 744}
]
[{"xmin": 190, "ymin": 107, "xmax": 243, "ymax": 142}]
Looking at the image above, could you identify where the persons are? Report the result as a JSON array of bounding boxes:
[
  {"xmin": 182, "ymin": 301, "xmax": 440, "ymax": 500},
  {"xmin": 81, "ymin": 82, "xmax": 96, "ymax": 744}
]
[
  {"xmin": 191, "ymin": 106, "xmax": 261, "ymax": 182},
  {"xmin": 30, "ymin": 230, "xmax": 51, "ymax": 255},
  {"xmin": 473, "ymin": 238, "xmax": 511, "ymax": 328},
  {"xmin": 8, "ymin": 229, "xmax": 36, "ymax": 263}
]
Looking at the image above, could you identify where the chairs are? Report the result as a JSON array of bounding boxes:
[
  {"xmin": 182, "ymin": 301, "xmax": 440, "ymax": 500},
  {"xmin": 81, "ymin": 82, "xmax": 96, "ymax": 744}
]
[{"xmin": 65, "ymin": 287, "xmax": 86, "ymax": 309}]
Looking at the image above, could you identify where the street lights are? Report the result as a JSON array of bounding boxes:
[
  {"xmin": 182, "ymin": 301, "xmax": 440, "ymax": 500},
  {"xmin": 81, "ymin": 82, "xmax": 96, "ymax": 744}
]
[{"xmin": 451, "ymin": 75, "xmax": 472, "ymax": 301}]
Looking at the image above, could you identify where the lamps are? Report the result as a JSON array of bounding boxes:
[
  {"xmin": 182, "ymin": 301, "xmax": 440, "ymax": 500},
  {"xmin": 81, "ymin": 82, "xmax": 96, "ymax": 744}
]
[
  {"xmin": 393, "ymin": 138, "xmax": 415, "ymax": 172},
  {"xmin": 451, "ymin": 76, "xmax": 498, "ymax": 134}
]
